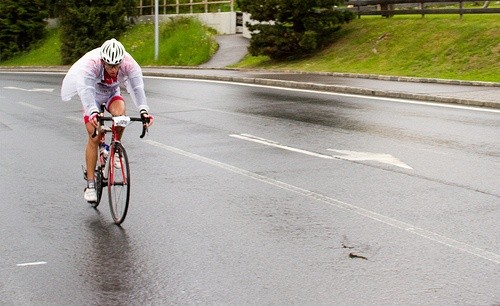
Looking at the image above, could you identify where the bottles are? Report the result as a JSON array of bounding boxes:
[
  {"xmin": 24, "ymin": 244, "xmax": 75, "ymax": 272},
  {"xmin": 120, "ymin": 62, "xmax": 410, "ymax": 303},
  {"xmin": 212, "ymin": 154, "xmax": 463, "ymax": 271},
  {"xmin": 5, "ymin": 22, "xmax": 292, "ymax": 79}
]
[{"xmin": 103, "ymin": 145, "xmax": 109, "ymax": 165}]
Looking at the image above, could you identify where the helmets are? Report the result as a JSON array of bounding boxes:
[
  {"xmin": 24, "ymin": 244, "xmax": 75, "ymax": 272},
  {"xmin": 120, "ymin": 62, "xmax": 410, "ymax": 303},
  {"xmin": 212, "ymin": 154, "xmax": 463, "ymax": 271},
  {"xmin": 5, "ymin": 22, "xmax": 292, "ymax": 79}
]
[{"xmin": 101, "ymin": 38, "xmax": 124, "ymax": 65}]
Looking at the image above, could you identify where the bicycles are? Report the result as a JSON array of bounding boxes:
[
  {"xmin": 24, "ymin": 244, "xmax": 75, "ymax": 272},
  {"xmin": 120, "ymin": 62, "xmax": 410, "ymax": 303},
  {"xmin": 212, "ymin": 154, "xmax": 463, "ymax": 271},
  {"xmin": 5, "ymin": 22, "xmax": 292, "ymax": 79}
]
[{"xmin": 80, "ymin": 103, "xmax": 149, "ymax": 226}]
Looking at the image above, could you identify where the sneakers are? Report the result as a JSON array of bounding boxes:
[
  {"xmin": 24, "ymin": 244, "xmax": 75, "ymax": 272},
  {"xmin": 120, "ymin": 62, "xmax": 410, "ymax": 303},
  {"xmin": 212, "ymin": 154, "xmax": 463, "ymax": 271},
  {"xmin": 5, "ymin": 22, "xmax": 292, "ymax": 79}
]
[
  {"xmin": 109, "ymin": 147, "xmax": 128, "ymax": 169},
  {"xmin": 83, "ymin": 180, "xmax": 102, "ymax": 205}
]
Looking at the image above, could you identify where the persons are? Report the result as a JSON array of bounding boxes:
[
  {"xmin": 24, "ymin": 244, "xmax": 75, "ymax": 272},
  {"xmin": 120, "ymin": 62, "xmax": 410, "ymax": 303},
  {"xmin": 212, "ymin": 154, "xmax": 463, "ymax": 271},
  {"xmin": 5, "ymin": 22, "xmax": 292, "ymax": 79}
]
[{"xmin": 61, "ymin": 38, "xmax": 154, "ymax": 203}]
